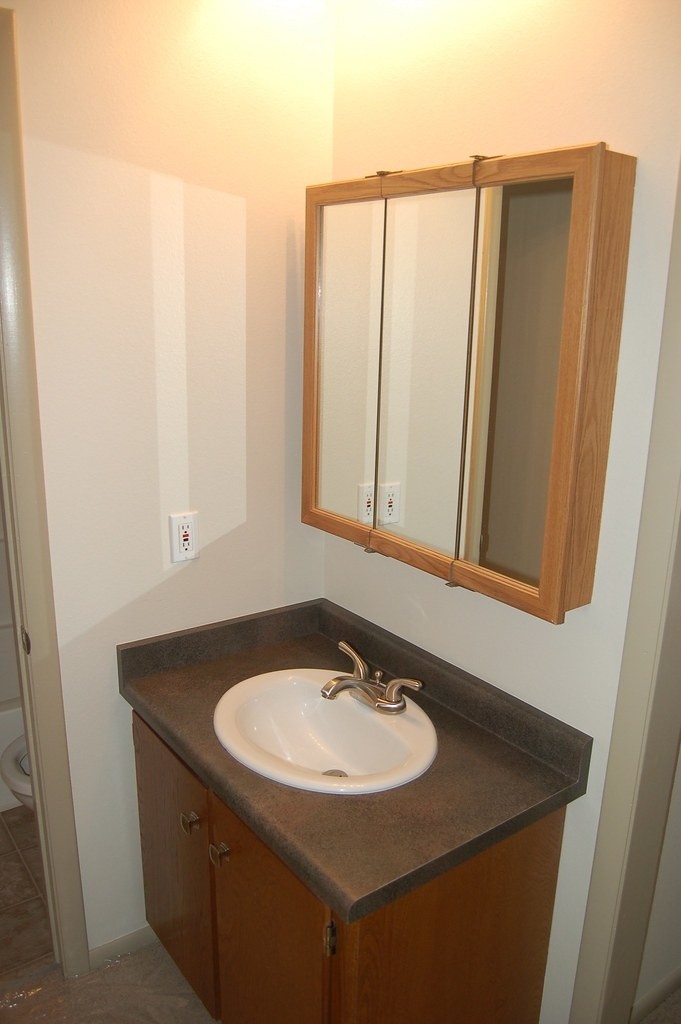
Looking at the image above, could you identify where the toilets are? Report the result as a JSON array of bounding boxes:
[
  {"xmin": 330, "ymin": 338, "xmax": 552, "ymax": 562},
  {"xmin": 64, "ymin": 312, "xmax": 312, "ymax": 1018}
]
[{"xmin": 0, "ymin": 736, "xmax": 36, "ymax": 813}]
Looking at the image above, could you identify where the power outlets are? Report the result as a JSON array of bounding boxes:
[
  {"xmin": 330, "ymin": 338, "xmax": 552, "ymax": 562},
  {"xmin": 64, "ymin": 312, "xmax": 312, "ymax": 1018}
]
[
  {"xmin": 167, "ymin": 508, "xmax": 200, "ymax": 563},
  {"xmin": 355, "ymin": 483, "xmax": 373, "ymax": 523},
  {"xmin": 379, "ymin": 481, "xmax": 402, "ymax": 527}
]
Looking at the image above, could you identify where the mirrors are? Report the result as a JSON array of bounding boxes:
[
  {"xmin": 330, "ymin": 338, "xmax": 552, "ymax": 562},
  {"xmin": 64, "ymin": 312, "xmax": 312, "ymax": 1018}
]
[{"xmin": 274, "ymin": 142, "xmax": 632, "ymax": 619}]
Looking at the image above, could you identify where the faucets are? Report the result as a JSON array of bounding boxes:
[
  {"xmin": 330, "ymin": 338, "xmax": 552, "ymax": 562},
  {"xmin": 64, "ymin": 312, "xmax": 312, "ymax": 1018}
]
[{"xmin": 320, "ymin": 670, "xmax": 385, "ymax": 708}]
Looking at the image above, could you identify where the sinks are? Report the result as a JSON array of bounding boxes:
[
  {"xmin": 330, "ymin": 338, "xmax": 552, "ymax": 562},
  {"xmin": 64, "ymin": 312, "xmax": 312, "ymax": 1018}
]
[{"xmin": 212, "ymin": 667, "xmax": 439, "ymax": 795}]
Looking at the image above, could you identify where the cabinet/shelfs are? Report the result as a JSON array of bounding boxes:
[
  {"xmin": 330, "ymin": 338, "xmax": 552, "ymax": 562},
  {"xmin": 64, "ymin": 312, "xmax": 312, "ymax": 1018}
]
[{"xmin": 130, "ymin": 705, "xmax": 569, "ymax": 1024}]
